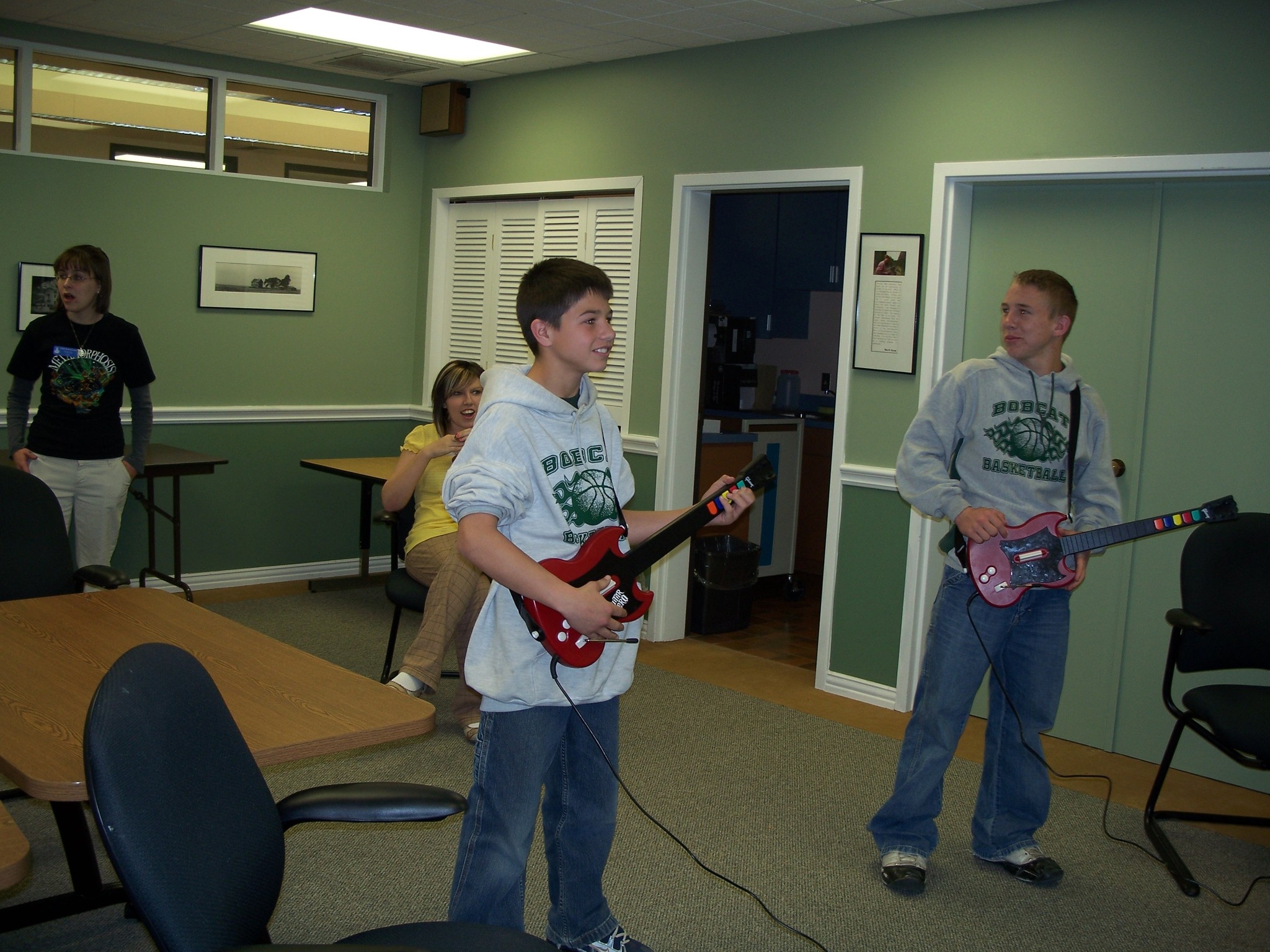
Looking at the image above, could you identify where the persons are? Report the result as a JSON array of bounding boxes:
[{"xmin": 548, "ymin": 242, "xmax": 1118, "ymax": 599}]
[
  {"xmin": 441, "ymin": 257, "xmax": 755, "ymax": 952},
  {"xmin": 6, "ymin": 244, "xmax": 156, "ymax": 592},
  {"xmin": 381, "ymin": 360, "xmax": 485, "ymax": 743},
  {"xmin": 868, "ymin": 269, "xmax": 1123, "ymax": 896}
]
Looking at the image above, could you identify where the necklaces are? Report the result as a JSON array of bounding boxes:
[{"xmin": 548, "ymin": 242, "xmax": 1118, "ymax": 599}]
[{"xmin": 70, "ymin": 319, "xmax": 94, "ymax": 357}]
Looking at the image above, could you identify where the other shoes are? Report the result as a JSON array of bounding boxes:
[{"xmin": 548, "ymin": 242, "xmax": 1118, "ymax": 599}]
[
  {"xmin": 463, "ymin": 725, "xmax": 480, "ymax": 740},
  {"xmin": 385, "ymin": 680, "xmax": 426, "ymax": 698}
]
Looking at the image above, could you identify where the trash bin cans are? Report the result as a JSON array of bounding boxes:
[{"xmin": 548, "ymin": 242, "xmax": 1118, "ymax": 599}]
[{"xmin": 685, "ymin": 534, "xmax": 761, "ymax": 634}]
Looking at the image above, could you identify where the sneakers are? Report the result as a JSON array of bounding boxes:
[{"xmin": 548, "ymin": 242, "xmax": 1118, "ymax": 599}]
[
  {"xmin": 881, "ymin": 852, "xmax": 927, "ymax": 893},
  {"xmin": 546, "ymin": 920, "xmax": 653, "ymax": 952},
  {"xmin": 996, "ymin": 845, "xmax": 1064, "ymax": 883}
]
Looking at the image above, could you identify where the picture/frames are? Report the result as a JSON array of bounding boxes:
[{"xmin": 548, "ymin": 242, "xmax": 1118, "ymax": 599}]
[
  {"xmin": 16, "ymin": 260, "xmax": 58, "ymax": 331},
  {"xmin": 198, "ymin": 245, "xmax": 318, "ymax": 312}
]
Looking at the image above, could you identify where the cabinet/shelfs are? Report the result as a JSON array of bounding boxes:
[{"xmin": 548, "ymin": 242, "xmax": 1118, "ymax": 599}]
[
  {"xmin": 704, "ymin": 417, "xmax": 833, "ymax": 577},
  {"xmin": 697, "ymin": 443, "xmax": 754, "ymax": 542}
]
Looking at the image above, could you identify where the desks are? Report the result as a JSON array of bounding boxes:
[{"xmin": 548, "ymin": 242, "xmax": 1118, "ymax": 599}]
[
  {"xmin": 0, "ymin": 444, "xmax": 229, "ymax": 605},
  {"xmin": 0, "ymin": 588, "xmax": 437, "ymax": 933},
  {"xmin": 300, "ymin": 457, "xmax": 400, "ymax": 593}
]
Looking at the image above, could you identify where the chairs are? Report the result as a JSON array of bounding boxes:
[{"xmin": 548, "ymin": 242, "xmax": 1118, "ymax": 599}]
[
  {"xmin": 83, "ymin": 642, "xmax": 561, "ymax": 952},
  {"xmin": 0, "ymin": 465, "xmax": 79, "ymax": 602},
  {"xmin": 373, "ymin": 491, "xmax": 493, "ymax": 684},
  {"xmin": 1145, "ymin": 512, "xmax": 1270, "ymax": 897}
]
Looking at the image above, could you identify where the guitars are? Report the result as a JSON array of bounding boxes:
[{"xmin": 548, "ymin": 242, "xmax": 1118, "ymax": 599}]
[
  {"xmin": 510, "ymin": 453, "xmax": 778, "ymax": 668},
  {"xmin": 966, "ymin": 495, "xmax": 1239, "ymax": 608}
]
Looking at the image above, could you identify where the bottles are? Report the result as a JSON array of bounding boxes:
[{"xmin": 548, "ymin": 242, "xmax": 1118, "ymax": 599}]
[{"xmin": 776, "ymin": 370, "xmax": 803, "ymax": 413}]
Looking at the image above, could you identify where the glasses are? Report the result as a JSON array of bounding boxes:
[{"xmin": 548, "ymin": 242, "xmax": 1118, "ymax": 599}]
[{"xmin": 55, "ymin": 274, "xmax": 96, "ymax": 282}]
